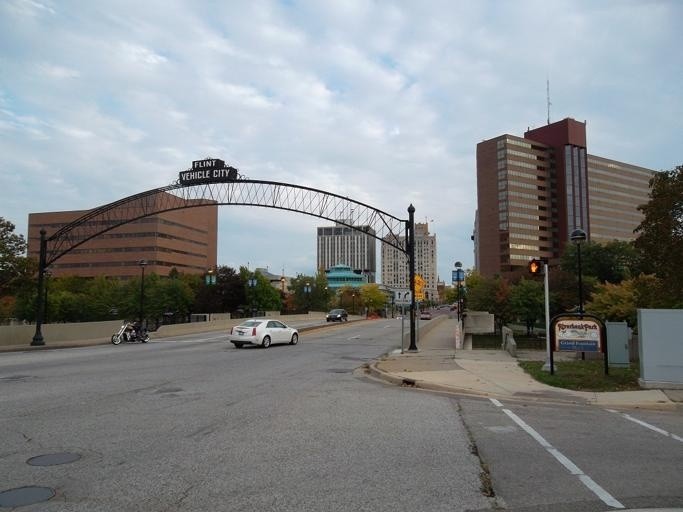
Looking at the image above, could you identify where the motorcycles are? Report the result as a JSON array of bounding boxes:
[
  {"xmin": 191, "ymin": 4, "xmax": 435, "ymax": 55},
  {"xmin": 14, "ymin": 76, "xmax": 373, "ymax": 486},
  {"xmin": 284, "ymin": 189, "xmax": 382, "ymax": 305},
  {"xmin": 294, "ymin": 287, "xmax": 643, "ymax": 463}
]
[{"xmin": 111, "ymin": 319, "xmax": 149, "ymax": 345}]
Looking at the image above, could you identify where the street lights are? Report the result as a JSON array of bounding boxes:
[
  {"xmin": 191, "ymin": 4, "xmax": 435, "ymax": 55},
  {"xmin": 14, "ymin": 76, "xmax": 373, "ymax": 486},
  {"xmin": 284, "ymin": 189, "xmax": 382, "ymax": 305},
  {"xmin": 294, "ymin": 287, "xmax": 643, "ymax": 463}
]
[
  {"xmin": 570, "ymin": 229, "xmax": 586, "ymax": 361},
  {"xmin": 305, "ymin": 280, "xmax": 310, "ymax": 313},
  {"xmin": 455, "ymin": 262, "xmax": 464, "ymax": 322},
  {"xmin": 208, "ymin": 265, "xmax": 215, "ymax": 322},
  {"xmin": 281, "ymin": 276, "xmax": 284, "ymax": 315},
  {"xmin": 138, "ymin": 259, "xmax": 147, "ymax": 321},
  {"xmin": 351, "ymin": 294, "xmax": 354, "ymax": 315},
  {"xmin": 338, "ymin": 289, "xmax": 343, "ymax": 309},
  {"xmin": 324, "ymin": 286, "xmax": 327, "ymax": 312}
]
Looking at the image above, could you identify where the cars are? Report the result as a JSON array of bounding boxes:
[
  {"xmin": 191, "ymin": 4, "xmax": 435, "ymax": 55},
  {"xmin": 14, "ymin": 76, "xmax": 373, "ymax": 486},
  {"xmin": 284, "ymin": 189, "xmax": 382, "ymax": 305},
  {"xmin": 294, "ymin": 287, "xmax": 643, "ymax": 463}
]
[
  {"xmin": 230, "ymin": 319, "xmax": 299, "ymax": 348},
  {"xmin": 419, "ymin": 311, "xmax": 432, "ymax": 320},
  {"xmin": 325, "ymin": 309, "xmax": 348, "ymax": 322}
]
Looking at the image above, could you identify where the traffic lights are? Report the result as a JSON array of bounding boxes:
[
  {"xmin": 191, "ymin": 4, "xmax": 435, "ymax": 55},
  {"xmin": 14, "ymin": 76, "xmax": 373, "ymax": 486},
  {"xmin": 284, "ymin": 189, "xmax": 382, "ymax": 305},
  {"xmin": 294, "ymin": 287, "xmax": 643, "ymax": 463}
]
[{"xmin": 528, "ymin": 260, "xmax": 541, "ymax": 274}]
